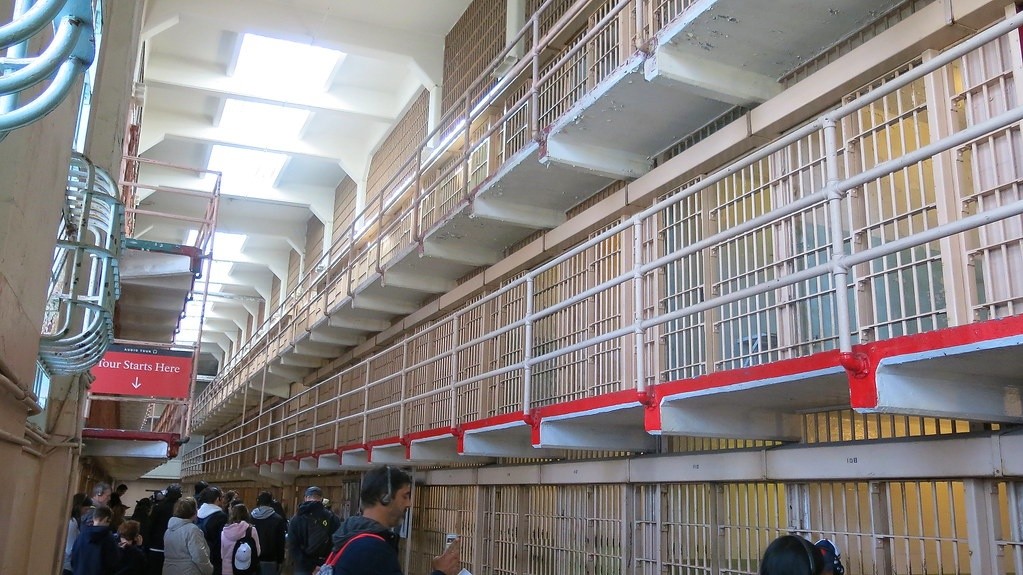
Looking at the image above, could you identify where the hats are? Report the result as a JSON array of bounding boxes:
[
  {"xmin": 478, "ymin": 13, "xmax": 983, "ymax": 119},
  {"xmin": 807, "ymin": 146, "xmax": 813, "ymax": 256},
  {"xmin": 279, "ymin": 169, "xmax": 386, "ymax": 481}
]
[
  {"xmin": 304, "ymin": 486, "xmax": 323, "ymax": 496},
  {"xmin": 813, "ymin": 538, "xmax": 841, "ymax": 560},
  {"xmin": 167, "ymin": 483, "xmax": 182, "ymax": 493}
]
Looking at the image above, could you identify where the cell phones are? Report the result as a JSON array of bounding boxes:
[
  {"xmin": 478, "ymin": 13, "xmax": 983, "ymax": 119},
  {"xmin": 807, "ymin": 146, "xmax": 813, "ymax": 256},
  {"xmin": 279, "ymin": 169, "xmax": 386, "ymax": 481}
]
[{"xmin": 445, "ymin": 533, "xmax": 458, "ymax": 552}]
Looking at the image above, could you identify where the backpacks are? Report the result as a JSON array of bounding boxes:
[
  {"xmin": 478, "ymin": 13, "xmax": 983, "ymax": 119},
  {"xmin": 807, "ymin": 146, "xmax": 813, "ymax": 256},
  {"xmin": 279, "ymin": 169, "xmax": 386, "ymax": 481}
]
[
  {"xmin": 313, "ymin": 532, "xmax": 386, "ymax": 575},
  {"xmin": 232, "ymin": 526, "xmax": 261, "ymax": 575}
]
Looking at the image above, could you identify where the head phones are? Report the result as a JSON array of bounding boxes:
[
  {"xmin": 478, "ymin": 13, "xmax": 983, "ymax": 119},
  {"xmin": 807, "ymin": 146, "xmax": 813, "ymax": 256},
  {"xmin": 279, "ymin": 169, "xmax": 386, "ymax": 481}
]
[
  {"xmin": 210, "ymin": 486, "xmax": 222, "ymax": 501},
  {"xmin": 381, "ymin": 464, "xmax": 393, "ymax": 507},
  {"xmin": 96, "ymin": 481, "xmax": 103, "ymax": 495},
  {"xmin": 74, "ymin": 493, "xmax": 88, "ymax": 512}
]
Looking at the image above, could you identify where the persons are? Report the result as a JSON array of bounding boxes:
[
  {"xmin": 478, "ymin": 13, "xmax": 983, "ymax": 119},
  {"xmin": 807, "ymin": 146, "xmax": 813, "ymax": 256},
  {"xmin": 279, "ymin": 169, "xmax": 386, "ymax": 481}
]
[
  {"xmin": 194, "ymin": 486, "xmax": 227, "ymax": 575},
  {"xmin": 757, "ymin": 534, "xmax": 845, "ymax": 575},
  {"xmin": 329, "ymin": 465, "xmax": 462, "ymax": 575},
  {"xmin": 71, "ymin": 503, "xmax": 118, "ymax": 575},
  {"xmin": 220, "ymin": 503, "xmax": 261, "ymax": 575},
  {"xmin": 61, "ymin": 492, "xmax": 93, "ymax": 575},
  {"xmin": 116, "ymin": 520, "xmax": 154, "ymax": 575},
  {"xmin": 79, "ymin": 480, "xmax": 112, "ymax": 532},
  {"xmin": 162, "ymin": 496, "xmax": 214, "ymax": 575},
  {"xmin": 107, "ymin": 480, "xmax": 291, "ymax": 546},
  {"xmin": 285, "ymin": 486, "xmax": 341, "ymax": 575},
  {"xmin": 250, "ymin": 490, "xmax": 287, "ymax": 575}
]
